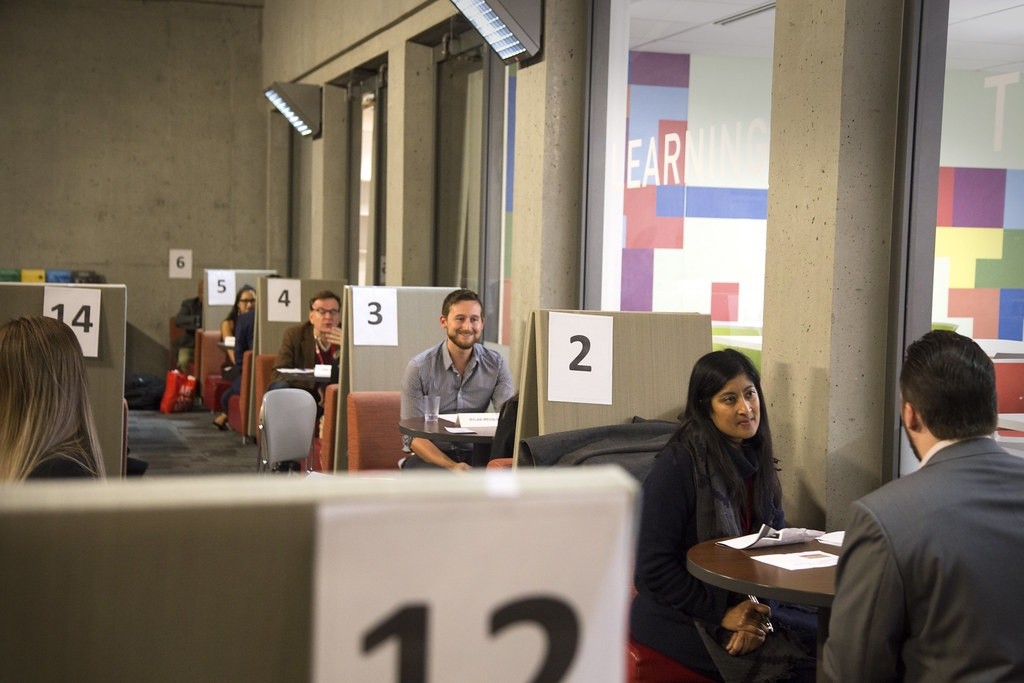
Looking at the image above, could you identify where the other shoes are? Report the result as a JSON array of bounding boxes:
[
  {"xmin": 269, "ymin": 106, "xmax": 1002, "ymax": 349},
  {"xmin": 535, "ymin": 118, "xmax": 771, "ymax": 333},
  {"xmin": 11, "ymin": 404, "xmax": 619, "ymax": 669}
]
[
  {"xmin": 272, "ymin": 459, "xmax": 302, "ymax": 472},
  {"xmin": 214, "ymin": 415, "xmax": 225, "ymax": 428}
]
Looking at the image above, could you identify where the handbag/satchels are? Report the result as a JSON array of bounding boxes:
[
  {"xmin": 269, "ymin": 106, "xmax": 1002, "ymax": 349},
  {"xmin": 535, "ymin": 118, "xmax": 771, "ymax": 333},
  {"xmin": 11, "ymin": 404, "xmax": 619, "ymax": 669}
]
[{"xmin": 160, "ymin": 369, "xmax": 198, "ymax": 415}]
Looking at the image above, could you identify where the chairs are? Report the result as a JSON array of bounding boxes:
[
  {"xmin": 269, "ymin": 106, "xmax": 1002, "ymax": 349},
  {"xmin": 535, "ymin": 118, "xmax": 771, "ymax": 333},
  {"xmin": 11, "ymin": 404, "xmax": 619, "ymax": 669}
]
[{"xmin": 0, "ymin": 269, "xmax": 712, "ymax": 682}]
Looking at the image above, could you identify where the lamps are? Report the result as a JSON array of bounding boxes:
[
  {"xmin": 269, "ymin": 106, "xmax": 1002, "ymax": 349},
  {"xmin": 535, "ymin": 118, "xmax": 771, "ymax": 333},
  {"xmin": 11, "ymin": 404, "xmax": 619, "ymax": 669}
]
[
  {"xmin": 452, "ymin": 0, "xmax": 544, "ymax": 65},
  {"xmin": 264, "ymin": 81, "xmax": 321, "ymax": 139}
]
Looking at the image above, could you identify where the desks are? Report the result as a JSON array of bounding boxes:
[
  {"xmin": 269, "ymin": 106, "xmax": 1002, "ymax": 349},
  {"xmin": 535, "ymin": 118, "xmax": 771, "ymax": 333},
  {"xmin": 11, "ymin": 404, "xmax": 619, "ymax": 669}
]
[
  {"xmin": 272, "ymin": 367, "xmax": 339, "ymax": 392},
  {"xmin": 686, "ymin": 536, "xmax": 849, "ymax": 683},
  {"xmin": 398, "ymin": 411, "xmax": 501, "ymax": 471}
]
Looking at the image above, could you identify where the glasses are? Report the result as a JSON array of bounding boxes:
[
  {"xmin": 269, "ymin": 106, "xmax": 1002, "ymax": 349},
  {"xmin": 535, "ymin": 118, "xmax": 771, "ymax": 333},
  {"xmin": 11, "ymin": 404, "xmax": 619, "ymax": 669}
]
[
  {"xmin": 239, "ymin": 299, "xmax": 255, "ymax": 304},
  {"xmin": 312, "ymin": 308, "xmax": 340, "ymax": 315}
]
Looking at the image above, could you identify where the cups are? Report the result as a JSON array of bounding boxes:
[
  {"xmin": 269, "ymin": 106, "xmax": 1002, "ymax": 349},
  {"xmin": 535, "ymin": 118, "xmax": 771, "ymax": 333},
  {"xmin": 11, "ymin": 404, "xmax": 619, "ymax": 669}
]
[{"xmin": 424, "ymin": 396, "xmax": 440, "ymax": 422}]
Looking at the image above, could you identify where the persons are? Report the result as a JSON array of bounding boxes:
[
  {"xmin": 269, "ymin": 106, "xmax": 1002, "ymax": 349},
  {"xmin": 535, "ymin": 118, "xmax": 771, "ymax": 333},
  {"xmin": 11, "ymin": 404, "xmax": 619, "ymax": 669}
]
[
  {"xmin": 399, "ymin": 289, "xmax": 514, "ymax": 472},
  {"xmin": 174, "ymin": 281, "xmax": 204, "ymax": 374},
  {"xmin": 0, "ymin": 318, "xmax": 104, "ymax": 479},
  {"xmin": 212, "ymin": 284, "xmax": 255, "ymax": 429},
  {"xmin": 631, "ymin": 348, "xmax": 821, "ymax": 683},
  {"xmin": 825, "ymin": 330, "xmax": 1023, "ymax": 683},
  {"xmin": 270, "ymin": 291, "xmax": 342, "ymax": 474}
]
